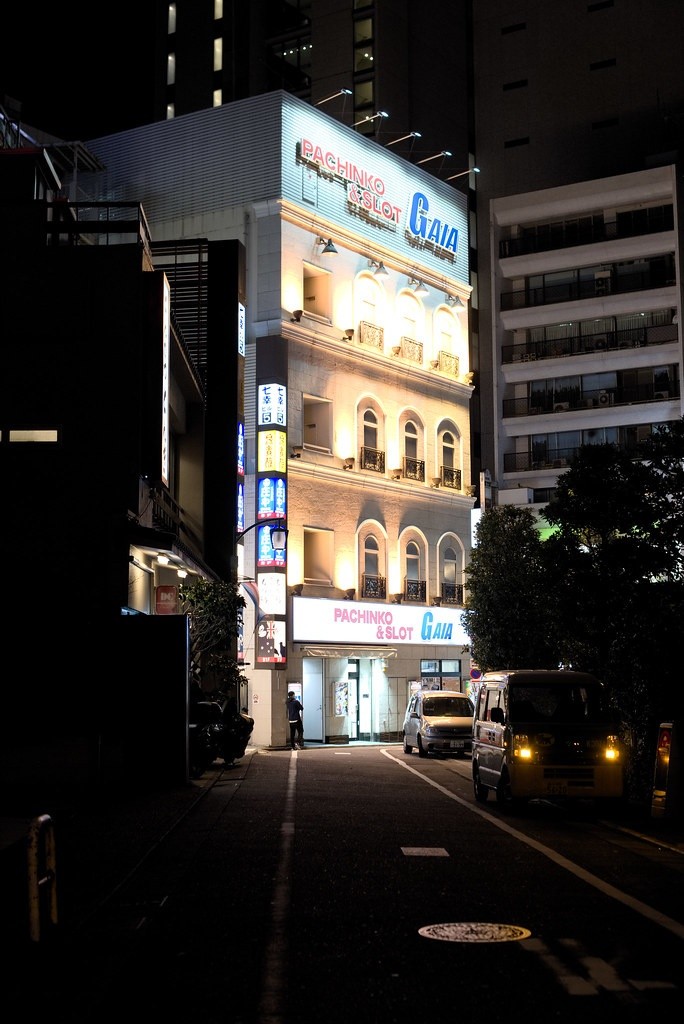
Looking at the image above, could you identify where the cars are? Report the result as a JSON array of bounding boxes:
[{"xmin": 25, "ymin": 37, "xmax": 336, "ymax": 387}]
[{"xmin": 401, "ymin": 691, "xmax": 476, "ymax": 758}]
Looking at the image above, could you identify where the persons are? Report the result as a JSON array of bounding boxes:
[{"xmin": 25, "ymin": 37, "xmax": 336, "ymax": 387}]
[{"xmin": 286, "ymin": 691, "xmax": 308, "ymax": 752}]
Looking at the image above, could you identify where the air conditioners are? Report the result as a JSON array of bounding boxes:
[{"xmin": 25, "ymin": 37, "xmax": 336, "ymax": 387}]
[
  {"xmin": 654, "ymin": 391, "xmax": 669, "ymax": 399},
  {"xmin": 554, "ymin": 402, "xmax": 570, "ymax": 412},
  {"xmin": 532, "ymin": 461, "xmax": 545, "ymax": 471},
  {"xmin": 618, "ymin": 340, "xmax": 632, "ymax": 350},
  {"xmin": 553, "ymin": 459, "xmax": 567, "ymax": 467},
  {"xmin": 592, "ymin": 334, "xmax": 609, "ymax": 349},
  {"xmin": 598, "ymin": 393, "xmax": 615, "ymax": 405},
  {"xmin": 576, "ymin": 399, "xmax": 593, "ymax": 408},
  {"xmin": 594, "ymin": 278, "xmax": 611, "ymax": 294}
]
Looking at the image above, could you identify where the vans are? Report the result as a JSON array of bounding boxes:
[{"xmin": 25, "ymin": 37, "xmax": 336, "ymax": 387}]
[{"xmin": 472, "ymin": 669, "xmax": 628, "ymax": 812}]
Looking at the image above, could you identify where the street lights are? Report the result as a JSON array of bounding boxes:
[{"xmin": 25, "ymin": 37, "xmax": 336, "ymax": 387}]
[{"xmin": 235, "ymin": 517, "xmax": 289, "ymax": 551}]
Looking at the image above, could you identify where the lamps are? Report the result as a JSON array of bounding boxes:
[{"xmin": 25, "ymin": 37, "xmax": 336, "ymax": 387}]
[
  {"xmin": 431, "ymin": 597, "xmax": 442, "ymax": 607},
  {"xmin": 392, "ymin": 593, "xmax": 404, "ymax": 604},
  {"xmin": 391, "ymin": 469, "xmax": 402, "ymax": 479},
  {"xmin": 290, "ymin": 310, "xmax": 303, "ymax": 323},
  {"xmin": 343, "ymin": 328, "xmax": 355, "ymax": 340},
  {"xmin": 344, "ymin": 588, "xmax": 356, "ymax": 600},
  {"xmin": 430, "ymin": 360, "xmax": 439, "ymax": 370},
  {"xmin": 291, "ymin": 445, "xmax": 302, "ymax": 458},
  {"xmin": 315, "ymin": 237, "xmax": 338, "ymax": 256},
  {"xmin": 430, "ymin": 478, "xmax": 442, "ymax": 488},
  {"xmin": 368, "ymin": 259, "xmax": 389, "ymax": 276},
  {"xmin": 293, "ymin": 584, "xmax": 303, "ymax": 596},
  {"xmin": 466, "ymin": 484, "xmax": 477, "ymax": 495},
  {"xmin": 464, "ymin": 372, "xmax": 475, "ymax": 384},
  {"xmin": 444, "ymin": 293, "xmax": 464, "ymax": 308},
  {"xmin": 343, "ymin": 458, "xmax": 355, "ymax": 470},
  {"xmin": 408, "ymin": 276, "xmax": 428, "ymax": 294},
  {"xmin": 392, "ymin": 347, "xmax": 402, "ymax": 356}
]
